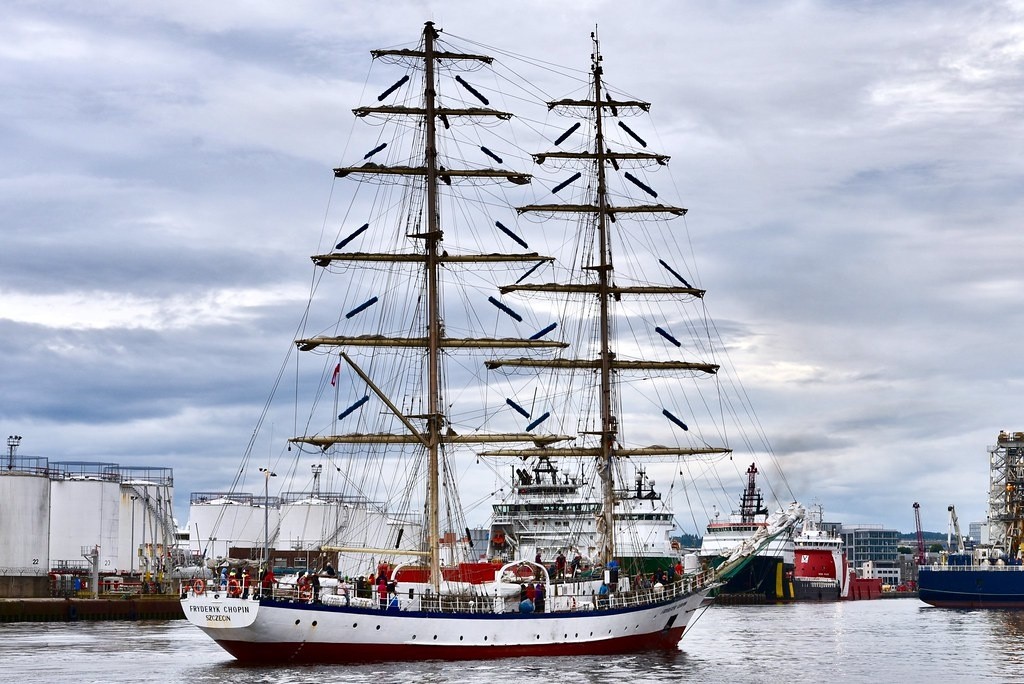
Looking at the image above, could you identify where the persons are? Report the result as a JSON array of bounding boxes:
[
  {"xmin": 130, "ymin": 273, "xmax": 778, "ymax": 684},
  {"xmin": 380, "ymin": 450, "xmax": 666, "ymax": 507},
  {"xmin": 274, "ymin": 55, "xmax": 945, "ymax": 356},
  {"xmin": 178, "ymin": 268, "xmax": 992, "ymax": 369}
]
[
  {"xmin": 376, "ymin": 571, "xmax": 387, "ymax": 585},
  {"xmin": 369, "ymin": 574, "xmax": 375, "ymax": 585},
  {"xmin": 556, "ymin": 554, "xmax": 567, "ymax": 579},
  {"xmin": 338, "ymin": 579, "xmax": 346, "ymax": 596},
  {"xmin": 535, "ymin": 552, "xmax": 543, "ymax": 581},
  {"xmin": 378, "ymin": 580, "xmax": 387, "ymax": 609},
  {"xmin": 297, "ymin": 572, "xmax": 320, "ymax": 599},
  {"xmin": 519, "ymin": 583, "xmax": 545, "ymax": 612},
  {"xmin": 358, "ymin": 576, "xmax": 366, "ymax": 598},
  {"xmin": 262, "ymin": 570, "xmax": 277, "ymax": 596},
  {"xmin": 599, "ymin": 584, "xmax": 608, "ymax": 605},
  {"xmin": 326, "ymin": 563, "xmax": 335, "ymax": 576},
  {"xmin": 387, "ymin": 580, "xmax": 397, "ymax": 598},
  {"xmin": 571, "ymin": 556, "xmax": 582, "ymax": 578},
  {"xmin": 242, "ymin": 569, "xmax": 250, "ymax": 598},
  {"xmin": 548, "ymin": 565, "xmax": 557, "ymax": 579},
  {"xmin": 227, "ymin": 575, "xmax": 242, "ymax": 598},
  {"xmin": 220, "ymin": 568, "xmax": 227, "ymax": 585},
  {"xmin": 634, "ymin": 561, "xmax": 684, "ymax": 588},
  {"xmin": 551, "ymin": 467, "xmax": 558, "ymax": 484}
]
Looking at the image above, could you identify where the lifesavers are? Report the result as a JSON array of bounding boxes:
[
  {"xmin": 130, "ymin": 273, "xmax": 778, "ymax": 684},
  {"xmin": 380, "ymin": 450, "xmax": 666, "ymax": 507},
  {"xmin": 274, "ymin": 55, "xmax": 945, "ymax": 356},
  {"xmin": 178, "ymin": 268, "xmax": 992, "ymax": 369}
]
[
  {"xmin": 300, "ymin": 585, "xmax": 312, "ymax": 600},
  {"xmin": 228, "ymin": 580, "xmax": 239, "ymax": 595},
  {"xmin": 193, "ymin": 578, "xmax": 205, "ymax": 595}
]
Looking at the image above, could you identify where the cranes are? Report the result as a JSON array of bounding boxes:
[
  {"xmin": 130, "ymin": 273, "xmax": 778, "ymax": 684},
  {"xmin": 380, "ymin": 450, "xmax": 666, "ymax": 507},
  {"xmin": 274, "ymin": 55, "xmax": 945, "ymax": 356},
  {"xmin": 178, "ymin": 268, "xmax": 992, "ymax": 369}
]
[
  {"xmin": 947, "ymin": 504, "xmax": 966, "ymax": 555},
  {"xmin": 913, "ymin": 501, "xmax": 924, "ymax": 565}
]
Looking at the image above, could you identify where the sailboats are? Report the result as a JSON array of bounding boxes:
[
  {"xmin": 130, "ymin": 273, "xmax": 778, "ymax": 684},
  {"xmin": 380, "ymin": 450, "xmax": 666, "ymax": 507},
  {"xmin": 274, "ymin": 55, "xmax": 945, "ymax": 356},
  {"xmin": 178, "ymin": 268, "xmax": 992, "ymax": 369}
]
[{"xmin": 181, "ymin": 18, "xmax": 810, "ymax": 671}]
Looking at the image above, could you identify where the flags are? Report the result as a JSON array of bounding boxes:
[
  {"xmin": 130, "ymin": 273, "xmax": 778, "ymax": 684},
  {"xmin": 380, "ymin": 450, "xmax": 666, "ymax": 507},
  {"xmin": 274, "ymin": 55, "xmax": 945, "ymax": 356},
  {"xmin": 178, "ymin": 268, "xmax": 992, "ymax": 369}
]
[{"xmin": 331, "ymin": 364, "xmax": 339, "ymax": 386}]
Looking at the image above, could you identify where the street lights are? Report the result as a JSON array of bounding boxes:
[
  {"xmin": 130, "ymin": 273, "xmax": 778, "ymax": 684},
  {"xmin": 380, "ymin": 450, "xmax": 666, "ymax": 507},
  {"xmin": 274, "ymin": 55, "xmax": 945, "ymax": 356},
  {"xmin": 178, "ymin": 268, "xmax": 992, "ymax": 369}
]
[{"xmin": 258, "ymin": 466, "xmax": 278, "ymax": 564}]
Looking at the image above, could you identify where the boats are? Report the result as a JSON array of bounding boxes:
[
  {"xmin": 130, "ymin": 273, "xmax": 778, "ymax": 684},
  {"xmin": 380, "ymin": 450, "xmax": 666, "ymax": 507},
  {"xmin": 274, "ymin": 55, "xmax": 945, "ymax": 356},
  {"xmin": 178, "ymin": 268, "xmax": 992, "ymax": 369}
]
[
  {"xmin": 699, "ymin": 463, "xmax": 849, "ymax": 605},
  {"xmin": 916, "ymin": 561, "xmax": 1024, "ymax": 610}
]
